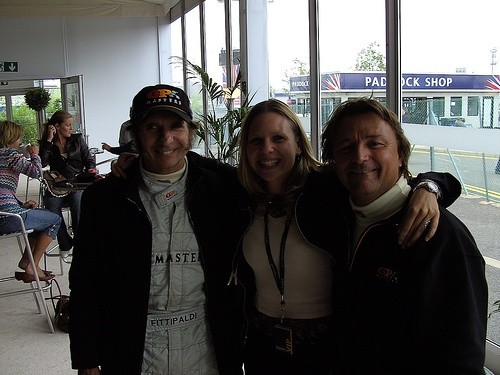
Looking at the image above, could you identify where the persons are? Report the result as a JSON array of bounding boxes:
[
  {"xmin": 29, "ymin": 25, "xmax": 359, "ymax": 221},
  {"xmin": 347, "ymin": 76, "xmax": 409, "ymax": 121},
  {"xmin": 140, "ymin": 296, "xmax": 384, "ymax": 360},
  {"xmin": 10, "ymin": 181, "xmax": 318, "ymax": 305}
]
[
  {"xmin": 37, "ymin": 110, "xmax": 96, "ymax": 263},
  {"xmin": 321, "ymin": 97, "xmax": 489, "ymax": 375},
  {"xmin": 102, "ymin": 108, "xmax": 137, "ymax": 159},
  {"xmin": 110, "ymin": 99, "xmax": 461, "ymax": 375},
  {"xmin": 69, "ymin": 85, "xmax": 238, "ymax": 375},
  {"xmin": 0, "ymin": 120, "xmax": 62, "ymax": 283}
]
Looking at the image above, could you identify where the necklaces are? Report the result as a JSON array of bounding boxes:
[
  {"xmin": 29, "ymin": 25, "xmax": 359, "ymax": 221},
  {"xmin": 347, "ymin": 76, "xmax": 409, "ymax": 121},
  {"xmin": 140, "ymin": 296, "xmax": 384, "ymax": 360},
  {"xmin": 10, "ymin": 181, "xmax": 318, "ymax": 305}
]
[{"xmin": 59, "ymin": 144, "xmax": 65, "ymax": 149}]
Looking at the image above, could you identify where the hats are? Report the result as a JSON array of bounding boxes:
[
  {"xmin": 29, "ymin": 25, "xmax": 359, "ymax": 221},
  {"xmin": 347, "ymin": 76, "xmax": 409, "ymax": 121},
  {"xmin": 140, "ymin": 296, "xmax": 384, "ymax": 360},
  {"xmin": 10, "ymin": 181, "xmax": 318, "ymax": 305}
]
[{"xmin": 131, "ymin": 84, "xmax": 192, "ymax": 126}]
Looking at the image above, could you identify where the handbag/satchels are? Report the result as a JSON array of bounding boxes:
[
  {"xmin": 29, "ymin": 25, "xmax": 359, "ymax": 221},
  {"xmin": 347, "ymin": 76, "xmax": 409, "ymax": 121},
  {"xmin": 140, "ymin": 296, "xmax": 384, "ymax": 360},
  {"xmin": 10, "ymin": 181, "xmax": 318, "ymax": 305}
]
[{"xmin": 45, "ymin": 294, "xmax": 71, "ymax": 333}]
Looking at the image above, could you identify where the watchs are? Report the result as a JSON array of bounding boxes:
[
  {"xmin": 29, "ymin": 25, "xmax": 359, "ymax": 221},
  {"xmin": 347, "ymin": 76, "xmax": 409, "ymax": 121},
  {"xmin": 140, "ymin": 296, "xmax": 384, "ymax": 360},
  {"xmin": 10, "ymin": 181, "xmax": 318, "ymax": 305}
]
[{"xmin": 412, "ymin": 180, "xmax": 440, "ymax": 200}]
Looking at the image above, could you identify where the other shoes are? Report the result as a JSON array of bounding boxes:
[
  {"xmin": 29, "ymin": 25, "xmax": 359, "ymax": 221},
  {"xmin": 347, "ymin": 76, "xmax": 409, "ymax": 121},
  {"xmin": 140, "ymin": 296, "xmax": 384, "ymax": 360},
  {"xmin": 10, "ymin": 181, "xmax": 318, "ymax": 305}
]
[
  {"xmin": 22, "ymin": 270, "xmax": 54, "ymax": 284},
  {"xmin": 15, "ymin": 266, "xmax": 52, "ymax": 281},
  {"xmin": 60, "ymin": 250, "xmax": 72, "ymax": 262}
]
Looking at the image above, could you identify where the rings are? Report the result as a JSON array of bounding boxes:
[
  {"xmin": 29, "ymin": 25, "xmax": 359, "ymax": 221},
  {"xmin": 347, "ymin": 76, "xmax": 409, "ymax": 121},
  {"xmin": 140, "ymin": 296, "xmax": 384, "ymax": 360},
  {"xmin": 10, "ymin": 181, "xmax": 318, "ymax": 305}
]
[{"xmin": 421, "ymin": 220, "xmax": 431, "ymax": 228}]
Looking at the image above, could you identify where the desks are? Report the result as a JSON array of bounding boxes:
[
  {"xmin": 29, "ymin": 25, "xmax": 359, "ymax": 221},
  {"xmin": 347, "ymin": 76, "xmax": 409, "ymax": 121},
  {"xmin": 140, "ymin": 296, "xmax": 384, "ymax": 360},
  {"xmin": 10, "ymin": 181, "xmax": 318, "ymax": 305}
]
[{"xmin": 52, "ymin": 179, "xmax": 92, "ymax": 189}]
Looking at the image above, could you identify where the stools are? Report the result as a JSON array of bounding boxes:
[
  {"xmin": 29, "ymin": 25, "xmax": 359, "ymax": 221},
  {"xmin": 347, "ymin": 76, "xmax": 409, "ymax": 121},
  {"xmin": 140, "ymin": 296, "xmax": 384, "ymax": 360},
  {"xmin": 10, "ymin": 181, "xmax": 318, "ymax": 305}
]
[
  {"xmin": 0, "ymin": 210, "xmax": 56, "ymax": 334},
  {"xmin": 37, "ymin": 179, "xmax": 72, "ymax": 275}
]
